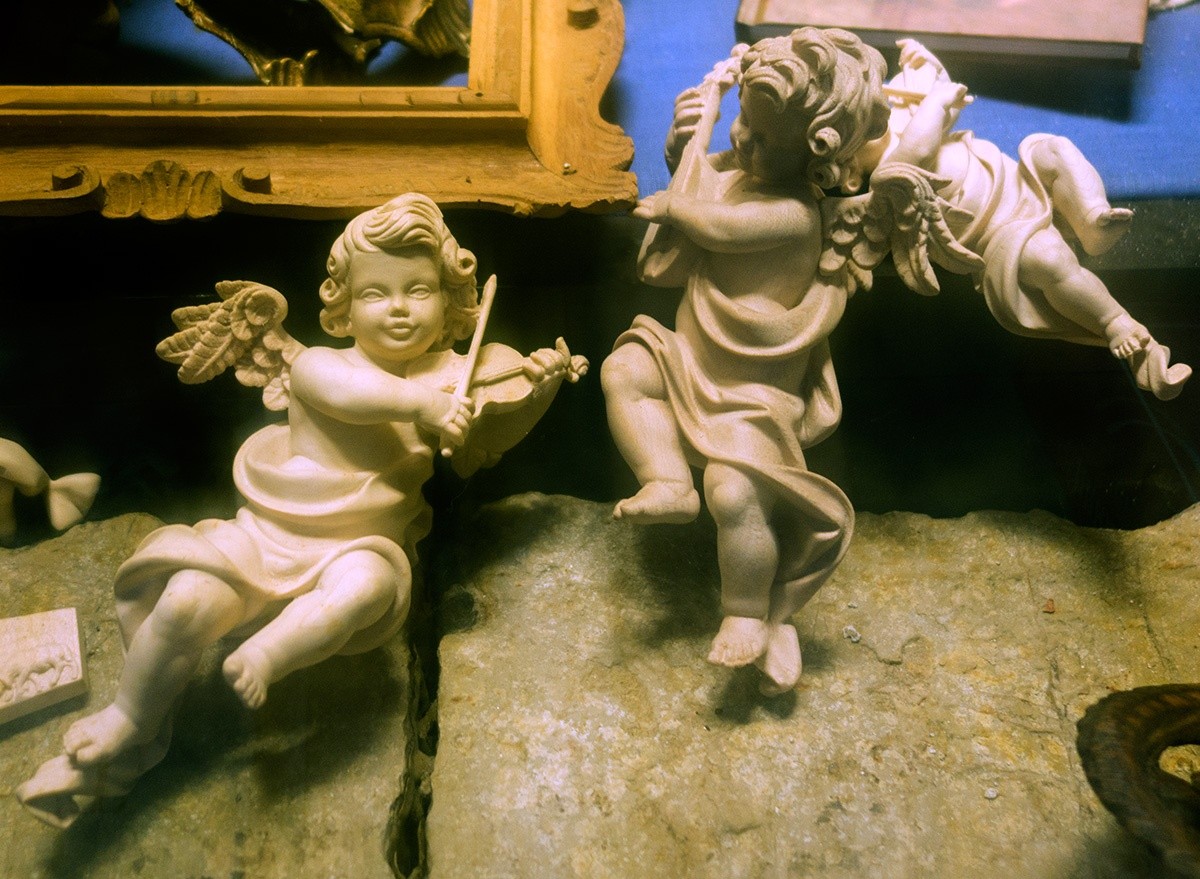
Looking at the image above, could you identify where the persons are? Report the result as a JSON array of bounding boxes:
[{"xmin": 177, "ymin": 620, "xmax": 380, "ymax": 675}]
[
  {"xmin": 59, "ymin": 190, "xmax": 588, "ymax": 772},
  {"xmin": 840, "ymin": 36, "xmax": 1151, "ymax": 360},
  {"xmin": 597, "ymin": 25, "xmax": 891, "ymax": 668}
]
